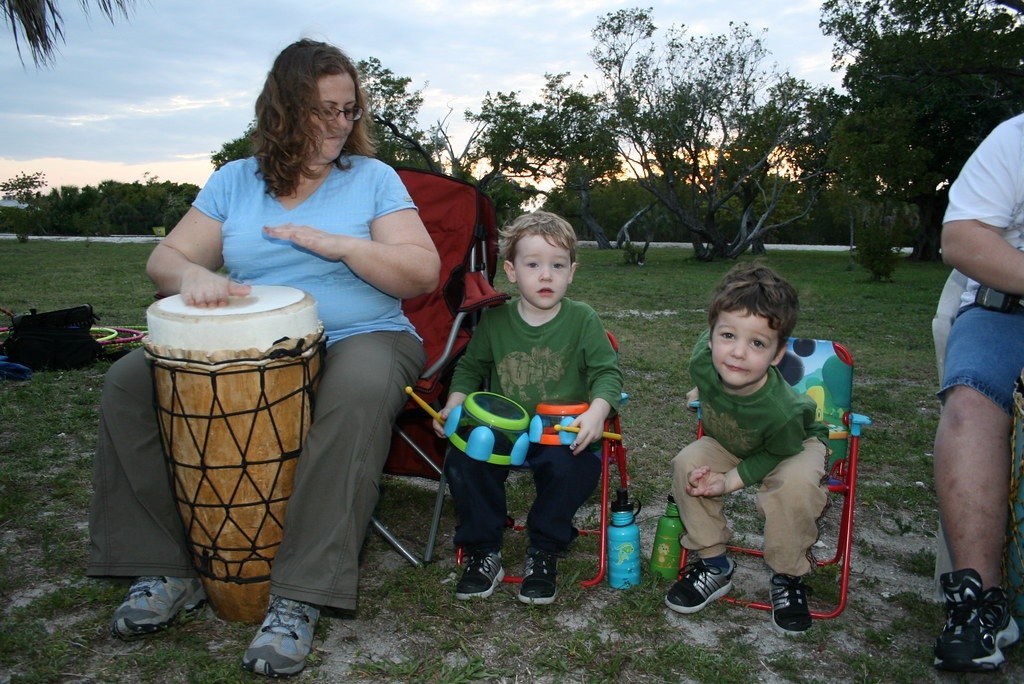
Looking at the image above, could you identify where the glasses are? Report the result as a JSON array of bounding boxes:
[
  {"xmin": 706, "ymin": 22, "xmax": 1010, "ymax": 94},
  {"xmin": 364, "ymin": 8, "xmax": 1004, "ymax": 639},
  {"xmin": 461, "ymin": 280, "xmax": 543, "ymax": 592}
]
[{"xmin": 311, "ymin": 106, "xmax": 364, "ymax": 120}]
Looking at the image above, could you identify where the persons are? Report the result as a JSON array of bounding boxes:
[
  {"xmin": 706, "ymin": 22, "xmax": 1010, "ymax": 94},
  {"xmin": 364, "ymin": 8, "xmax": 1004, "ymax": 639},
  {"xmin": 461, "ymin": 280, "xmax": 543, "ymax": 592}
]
[
  {"xmin": 431, "ymin": 212, "xmax": 624, "ymax": 605},
  {"xmin": 664, "ymin": 264, "xmax": 829, "ymax": 638},
  {"xmin": 933, "ymin": 113, "xmax": 1024, "ymax": 675},
  {"xmin": 84, "ymin": 39, "xmax": 442, "ymax": 677}
]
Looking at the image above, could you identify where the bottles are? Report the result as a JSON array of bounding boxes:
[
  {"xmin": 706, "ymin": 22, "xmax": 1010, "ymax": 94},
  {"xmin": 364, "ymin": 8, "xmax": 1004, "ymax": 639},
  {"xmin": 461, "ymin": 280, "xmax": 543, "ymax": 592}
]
[
  {"xmin": 649, "ymin": 492, "xmax": 685, "ymax": 581},
  {"xmin": 607, "ymin": 489, "xmax": 641, "ymax": 591}
]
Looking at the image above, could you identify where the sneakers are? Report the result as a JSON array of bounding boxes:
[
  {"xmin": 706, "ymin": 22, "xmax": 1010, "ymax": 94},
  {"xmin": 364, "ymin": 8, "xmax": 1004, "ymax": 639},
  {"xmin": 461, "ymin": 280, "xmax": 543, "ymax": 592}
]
[
  {"xmin": 933, "ymin": 569, "xmax": 1019, "ymax": 671},
  {"xmin": 455, "ymin": 550, "xmax": 504, "ymax": 600},
  {"xmin": 769, "ymin": 571, "xmax": 814, "ymax": 635},
  {"xmin": 110, "ymin": 574, "xmax": 208, "ymax": 640},
  {"xmin": 243, "ymin": 591, "xmax": 322, "ymax": 674},
  {"xmin": 517, "ymin": 545, "xmax": 567, "ymax": 604},
  {"xmin": 665, "ymin": 556, "xmax": 739, "ymax": 614}
]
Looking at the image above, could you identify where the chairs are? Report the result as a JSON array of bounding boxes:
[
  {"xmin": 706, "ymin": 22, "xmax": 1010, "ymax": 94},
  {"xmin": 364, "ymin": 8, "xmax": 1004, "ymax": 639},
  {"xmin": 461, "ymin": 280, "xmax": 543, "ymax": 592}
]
[
  {"xmin": 369, "ymin": 166, "xmax": 510, "ymax": 569},
  {"xmin": 456, "ymin": 328, "xmax": 633, "ymax": 590},
  {"xmin": 676, "ymin": 329, "xmax": 872, "ymax": 618},
  {"xmin": 932, "ymin": 264, "xmax": 1024, "ymax": 603}
]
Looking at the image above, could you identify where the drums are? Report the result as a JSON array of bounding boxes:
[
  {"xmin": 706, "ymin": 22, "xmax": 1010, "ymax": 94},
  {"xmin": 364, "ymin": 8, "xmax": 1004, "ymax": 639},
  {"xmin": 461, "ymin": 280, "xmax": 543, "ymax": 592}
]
[
  {"xmin": 443, "ymin": 390, "xmax": 531, "ymax": 466},
  {"xmin": 143, "ymin": 283, "xmax": 329, "ymax": 618},
  {"xmin": 1008, "ymin": 366, "xmax": 1024, "ymax": 641},
  {"xmin": 530, "ymin": 400, "xmax": 590, "ymax": 447}
]
